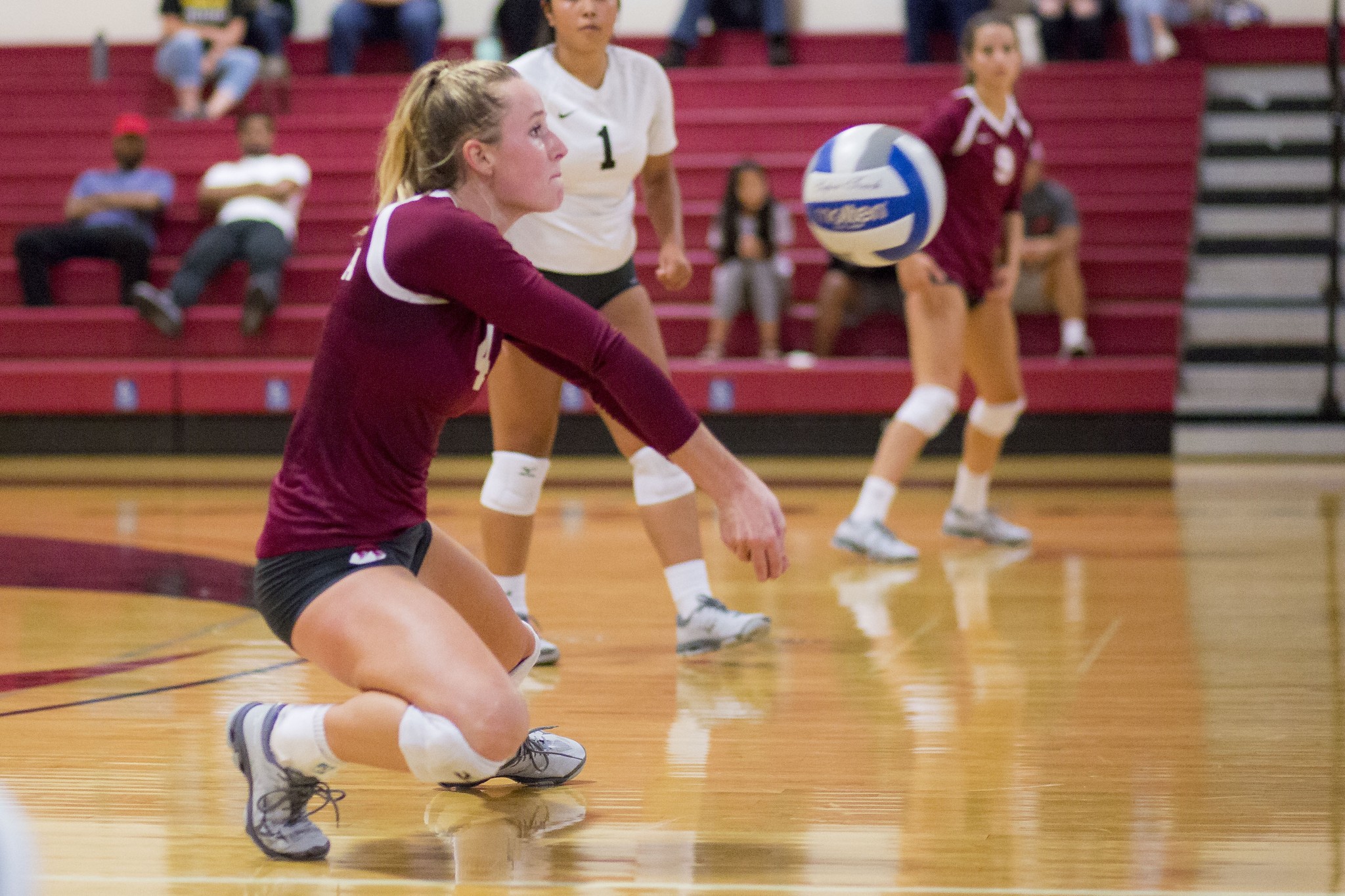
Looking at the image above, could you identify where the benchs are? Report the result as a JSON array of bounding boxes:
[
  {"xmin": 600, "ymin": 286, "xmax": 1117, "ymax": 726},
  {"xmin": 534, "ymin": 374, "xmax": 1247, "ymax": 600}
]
[{"xmin": 0, "ymin": 33, "xmax": 1214, "ymax": 454}]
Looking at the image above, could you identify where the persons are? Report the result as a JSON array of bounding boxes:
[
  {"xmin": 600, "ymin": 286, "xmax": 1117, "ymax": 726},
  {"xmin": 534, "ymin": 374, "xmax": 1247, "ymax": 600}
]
[
  {"xmin": 229, "ymin": 60, "xmax": 790, "ymax": 860},
  {"xmin": 813, "ymin": 261, "xmax": 902, "ymax": 357},
  {"xmin": 832, "ymin": 14, "xmax": 1045, "ymax": 566},
  {"xmin": 122, "ymin": 104, "xmax": 312, "ymax": 340},
  {"xmin": 1014, "ymin": 173, "xmax": 1094, "ymax": 360},
  {"xmin": 699, "ymin": 154, "xmax": 795, "ymax": 361},
  {"xmin": 479, "ymin": 0, "xmax": 776, "ymax": 665},
  {"xmin": 151, "ymin": 0, "xmax": 296, "ymax": 132},
  {"xmin": 329, "ymin": 0, "xmax": 443, "ymax": 75},
  {"xmin": 905, "ymin": 0, "xmax": 1260, "ymax": 61},
  {"xmin": 12, "ymin": 111, "xmax": 179, "ymax": 305}
]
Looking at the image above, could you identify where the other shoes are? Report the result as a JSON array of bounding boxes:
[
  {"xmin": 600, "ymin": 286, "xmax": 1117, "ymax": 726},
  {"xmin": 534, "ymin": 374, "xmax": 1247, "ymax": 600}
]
[
  {"xmin": 237, "ymin": 275, "xmax": 282, "ymax": 336},
  {"xmin": 132, "ymin": 282, "xmax": 186, "ymax": 337},
  {"xmin": 1151, "ymin": 35, "xmax": 1179, "ymax": 60},
  {"xmin": 1056, "ymin": 335, "xmax": 1091, "ymax": 360}
]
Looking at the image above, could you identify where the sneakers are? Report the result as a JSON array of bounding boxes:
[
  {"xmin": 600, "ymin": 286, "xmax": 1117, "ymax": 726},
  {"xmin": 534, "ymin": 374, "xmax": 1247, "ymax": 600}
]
[
  {"xmin": 424, "ymin": 790, "xmax": 586, "ymax": 839},
  {"xmin": 831, "ymin": 567, "xmax": 918, "ymax": 606},
  {"xmin": 516, "ymin": 667, "xmax": 560, "ymax": 695},
  {"xmin": 674, "ymin": 593, "xmax": 772, "ymax": 659},
  {"xmin": 830, "ymin": 519, "xmax": 919, "ymax": 564},
  {"xmin": 942, "ymin": 549, "xmax": 1031, "ymax": 583},
  {"xmin": 941, "ymin": 505, "xmax": 1031, "ymax": 548},
  {"xmin": 437, "ymin": 725, "xmax": 587, "ymax": 789},
  {"xmin": 227, "ymin": 700, "xmax": 331, "ymax": 860},
  {"xmin": 676, "ymin": 662, "xmax": 774, "ymax": 722},
  {"xmin": 515, "ymin": 612, "xmax": 559, "ymax": 664}
]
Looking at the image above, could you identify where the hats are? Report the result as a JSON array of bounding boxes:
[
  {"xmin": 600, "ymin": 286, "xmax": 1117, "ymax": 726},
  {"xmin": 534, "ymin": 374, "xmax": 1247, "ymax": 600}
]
[{"xmin": 114, "ymin": 114, "xmax": 145, "ymax": 135}]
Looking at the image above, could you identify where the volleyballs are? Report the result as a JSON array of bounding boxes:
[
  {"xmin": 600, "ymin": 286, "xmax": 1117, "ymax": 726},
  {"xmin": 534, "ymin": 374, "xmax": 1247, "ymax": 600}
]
[{"xmin": 801, "ymin": 124, "xmax": 946, "ymax": 267}]
